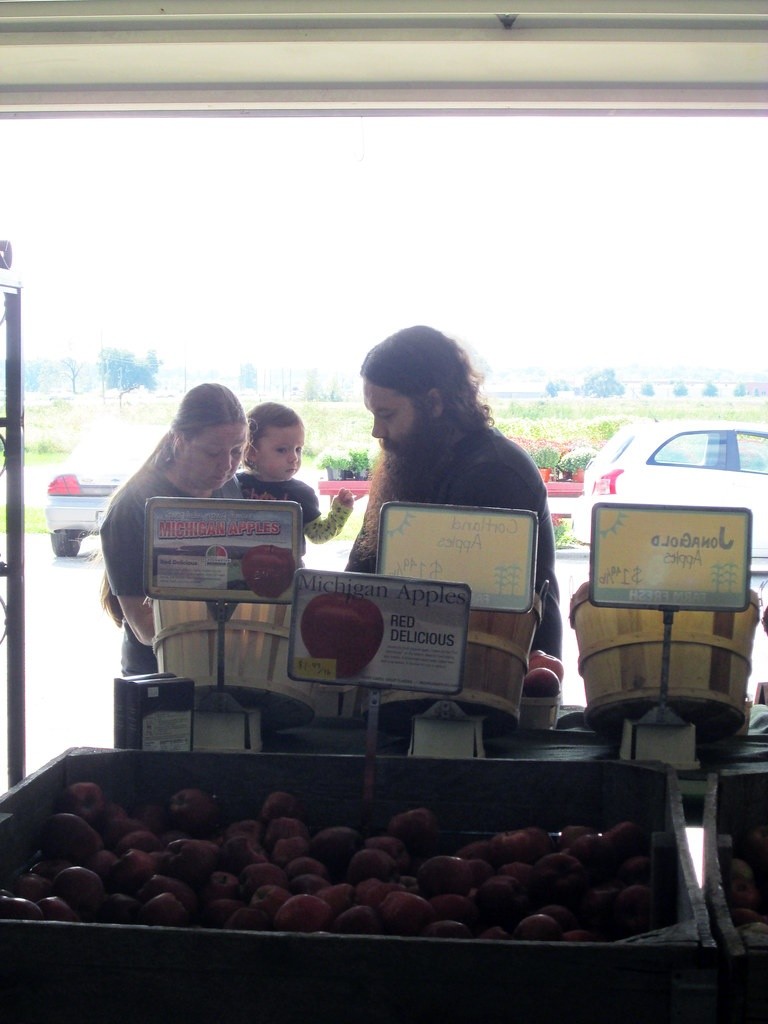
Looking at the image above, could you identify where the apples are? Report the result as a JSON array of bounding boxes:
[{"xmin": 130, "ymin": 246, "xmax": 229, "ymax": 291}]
[
  {"xmin": 521, "ymin": 650, "xmax": 563, "ymax": 698},
  {"xmin": 243, "ymin": 544, "xmax": 293, "ymax": 597},
  {"xmin": 301, "ymin": 592, "xmax": 384, "ymax": 677},
  {"xmin": 723, "ymin": 820, "xmax": 768, "ymax": 933},
  {"xmin": 0, "ymin": 780, "xmax": 659, "ymax": 942}
]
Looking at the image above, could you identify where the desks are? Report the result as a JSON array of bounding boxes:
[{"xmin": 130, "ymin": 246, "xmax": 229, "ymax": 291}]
[{"xmin": 266, "ymin": 705, "xmax": 768, "ymax": 827}]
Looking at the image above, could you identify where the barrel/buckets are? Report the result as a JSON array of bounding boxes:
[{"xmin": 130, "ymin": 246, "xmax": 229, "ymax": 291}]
[
  {"xmin": 360, "ymin": 578, "xmax": 544, "ymax": 729},
  {"xmin": 150, "ymin": 589, "xmax": 318, "ymax": 727},
  {"xmin": 569, "ymin": 579, "xmax": 760, "ymax": 743}
]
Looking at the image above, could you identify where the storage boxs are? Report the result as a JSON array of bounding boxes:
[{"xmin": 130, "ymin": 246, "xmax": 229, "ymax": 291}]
[
  {"xmin": 700, "ymin": 763, "xmax": 768, "ymax": 1024},
  {"xmin": 2, "ymin": 744, "xmax": 718, "ymax": 1024}
]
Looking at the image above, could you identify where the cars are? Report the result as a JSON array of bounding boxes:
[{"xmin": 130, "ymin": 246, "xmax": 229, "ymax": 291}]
[
  {"xmin": 571, "ymin": 418, "xmax": 768, "ymax": 573},
  {"xmin": 44, "ymin": 426, "xmax": 168, "ymax": 558}
]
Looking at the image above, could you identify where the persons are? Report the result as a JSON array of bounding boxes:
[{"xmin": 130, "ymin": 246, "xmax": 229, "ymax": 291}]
[
  {"xmin": 209, "ymin": 402, "xmax": 354, "ymax": 569},
  {"xmin": 345, "ymin": 326, "xmax": 562, "ymax": 662},
  {"xmin": 100, "ymin": 383, "xmax": 244, "ymax": 679}
]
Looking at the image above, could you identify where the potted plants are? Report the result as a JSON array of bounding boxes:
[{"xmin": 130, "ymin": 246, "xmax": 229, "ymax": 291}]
[
  {"xmin": 532, "ymin": 447, "xmax": 592, "ymax": 484},
  {"xmin": 318, "ymin": 449, "xmax": 371, "ymax": 481}
]
